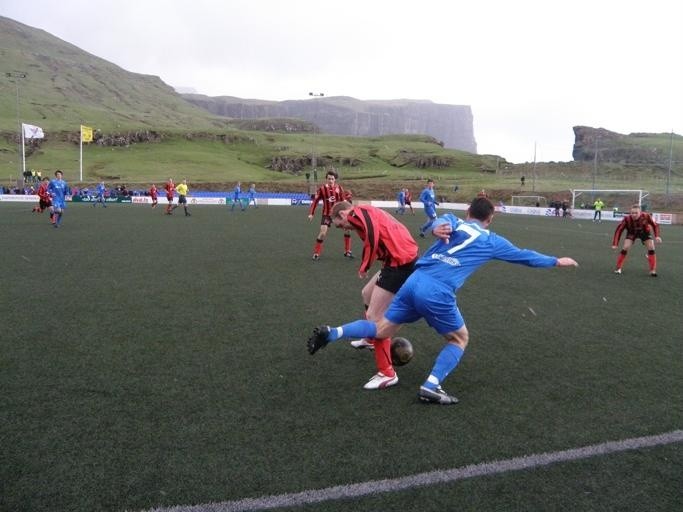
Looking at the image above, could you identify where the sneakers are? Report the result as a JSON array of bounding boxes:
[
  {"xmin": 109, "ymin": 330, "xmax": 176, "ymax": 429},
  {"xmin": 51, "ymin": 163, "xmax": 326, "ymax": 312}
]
[
  {"xmin": 349, "ymin": 337, "xmax": 376, "ymax": 353},
  {"xmin": 613, "ymin": 267, "xmax": 658, "ymax": 277},
  {"xmin": 419, "ymin": 226, "xmax": 425, "ymax": 238},
  {"xmin": 311, "ymin": 250, "xmax": 356, "ymax": 261},
  {"xmin": 305, "ymin": 322, "xmax": 331, "ymax": 354},
  {"xmin": 416, "ymin": 383, "xmax": 459, "ymax": 406},
  {"xmin": 361, "ymin": 371, "xmax": 402, "ymax": 389}
]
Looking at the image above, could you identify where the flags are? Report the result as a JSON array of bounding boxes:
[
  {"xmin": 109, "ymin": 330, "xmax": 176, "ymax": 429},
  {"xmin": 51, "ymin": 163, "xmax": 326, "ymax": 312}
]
[
  {"xmin": 82, "ymin": 125, "xmax": 93, "ymax": 142},
  {"xmin": 23, "ymin": 124, "xmax": 44, "ymax": 138}
]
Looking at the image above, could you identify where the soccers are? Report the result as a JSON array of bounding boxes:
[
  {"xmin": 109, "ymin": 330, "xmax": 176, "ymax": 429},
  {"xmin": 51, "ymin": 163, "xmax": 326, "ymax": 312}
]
[{"xmin": 390, "ymin": 337, "xmax": 413, "ymax": 366}]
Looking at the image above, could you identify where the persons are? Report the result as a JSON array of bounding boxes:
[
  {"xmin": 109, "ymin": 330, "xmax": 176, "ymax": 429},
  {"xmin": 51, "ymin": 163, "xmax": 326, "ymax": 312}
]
[
  {"xmin": 308, "ymin": 170, "xmax": 439, "ymax": 261},
  {"xmin": 476, "ymin": 188, "xmax": 488, "ymax": 199},
  {"xmin": 231, "ymin": 181, "xmax": 245, "ymax": 211},
  {"xmin": 307, "ymin": 198, "xmax": 579, "ymax": 405},
  {"xmin": 612, "ymin": 204, "xmax": 662, "ymax": 277},
  {"xmin": 246, "ymin": 183, "xmax": 258, "ymax": 208},
  {"xmin": 31, "ymin": 170, "xmax": 191, "ymax": 229},
  {"xmin": 329, "ymin": 201, "xmax": 419, "ymax": 390},
  {"xmin": 520, "ymin": 175, "xmax": 526, "ymax": 187},
  {"xmin": 536, "ymin": 197, "xmax": 605, "ymax": 222}
]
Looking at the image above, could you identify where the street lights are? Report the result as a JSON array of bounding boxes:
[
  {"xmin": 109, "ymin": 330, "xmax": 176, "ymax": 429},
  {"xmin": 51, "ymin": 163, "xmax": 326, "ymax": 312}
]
[
  {"xmin": 307, "ymin": 90, "xmax": 326, "ymax": 197},
  {"xmin": 5, "ymin": 70, "xmax": 26, "ymax": 179}
]
